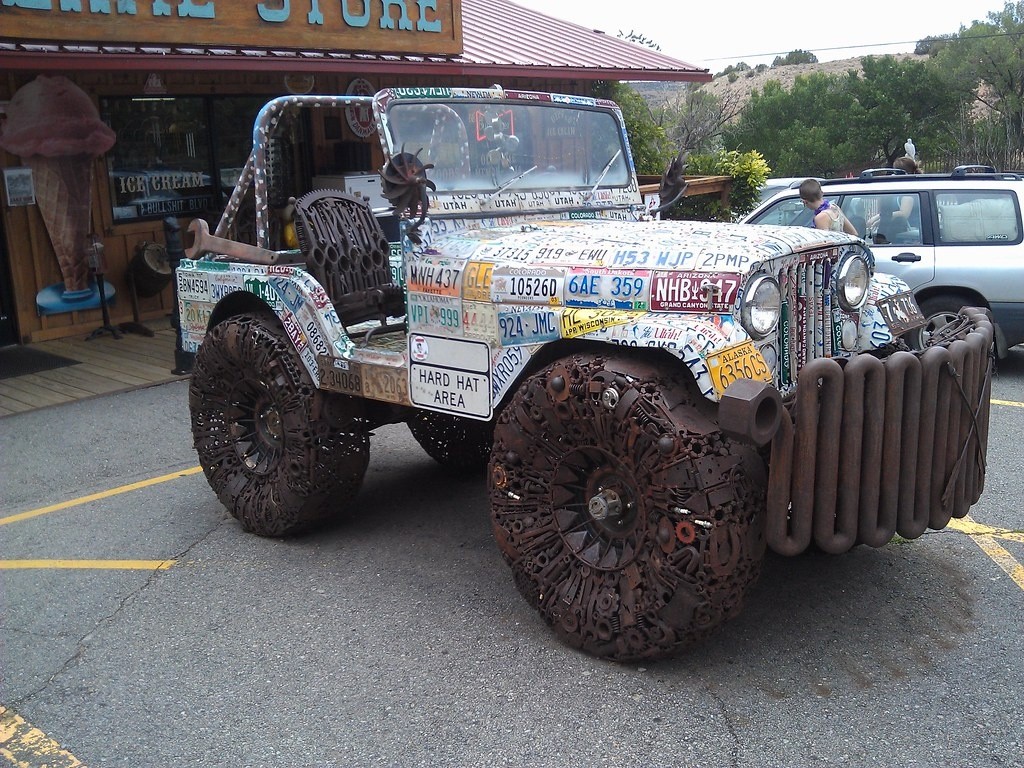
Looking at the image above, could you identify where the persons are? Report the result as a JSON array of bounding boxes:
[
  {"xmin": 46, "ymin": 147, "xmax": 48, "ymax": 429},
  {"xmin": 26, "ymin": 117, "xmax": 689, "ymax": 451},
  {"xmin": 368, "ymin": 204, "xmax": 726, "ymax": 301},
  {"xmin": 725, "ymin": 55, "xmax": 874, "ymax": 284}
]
[
  {"xmin": 799, "ymin": 179, "xmax": 859, "ymax": 236},
  {"xmin": 865, "ymin": 157, "xmax": 922, "ymax": 244}
]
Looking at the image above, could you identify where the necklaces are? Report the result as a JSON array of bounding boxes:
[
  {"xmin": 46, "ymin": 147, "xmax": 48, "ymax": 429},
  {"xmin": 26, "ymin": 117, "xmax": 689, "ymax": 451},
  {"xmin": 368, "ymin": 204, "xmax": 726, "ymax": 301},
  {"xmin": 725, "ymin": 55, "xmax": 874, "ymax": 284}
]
[{"xmin": 811, "ymin": 199, "xmax": 830, "ymax": 228}]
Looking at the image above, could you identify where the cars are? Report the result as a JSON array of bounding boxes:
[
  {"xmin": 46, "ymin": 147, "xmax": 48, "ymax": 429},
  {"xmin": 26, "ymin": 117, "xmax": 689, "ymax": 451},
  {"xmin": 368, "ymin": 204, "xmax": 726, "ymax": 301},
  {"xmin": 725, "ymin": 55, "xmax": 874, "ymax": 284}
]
[{"xmin": 738, "ymin": 177, "xmax": 864, "ymax": 224}]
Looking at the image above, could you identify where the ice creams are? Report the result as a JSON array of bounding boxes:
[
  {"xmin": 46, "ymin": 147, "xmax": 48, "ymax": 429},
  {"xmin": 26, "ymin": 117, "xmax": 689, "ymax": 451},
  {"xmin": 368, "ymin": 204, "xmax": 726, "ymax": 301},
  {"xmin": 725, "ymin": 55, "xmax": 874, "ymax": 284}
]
[{"xmin": 2, "ymin": 74, "xmax": 116, "ymax": 292}]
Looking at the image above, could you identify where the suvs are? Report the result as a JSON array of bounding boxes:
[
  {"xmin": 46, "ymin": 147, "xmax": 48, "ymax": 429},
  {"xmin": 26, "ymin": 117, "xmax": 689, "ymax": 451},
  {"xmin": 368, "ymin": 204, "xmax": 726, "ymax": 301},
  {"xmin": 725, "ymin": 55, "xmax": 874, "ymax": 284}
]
[
  {"xmin": 177, "ymin": 87, "xmax": 990, "ymax": 670},
  {"xmin": 738, "ymin": 160, "xmax": 1024, "ymax": 360}
]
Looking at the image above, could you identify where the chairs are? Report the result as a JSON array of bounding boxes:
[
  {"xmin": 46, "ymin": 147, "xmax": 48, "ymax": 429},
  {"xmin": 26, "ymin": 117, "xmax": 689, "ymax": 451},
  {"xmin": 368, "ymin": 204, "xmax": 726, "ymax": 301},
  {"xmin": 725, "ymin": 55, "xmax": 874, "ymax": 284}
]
[{"xmin": 296, "ymin": 189, "xmax": 405, "ymax": 327}]
